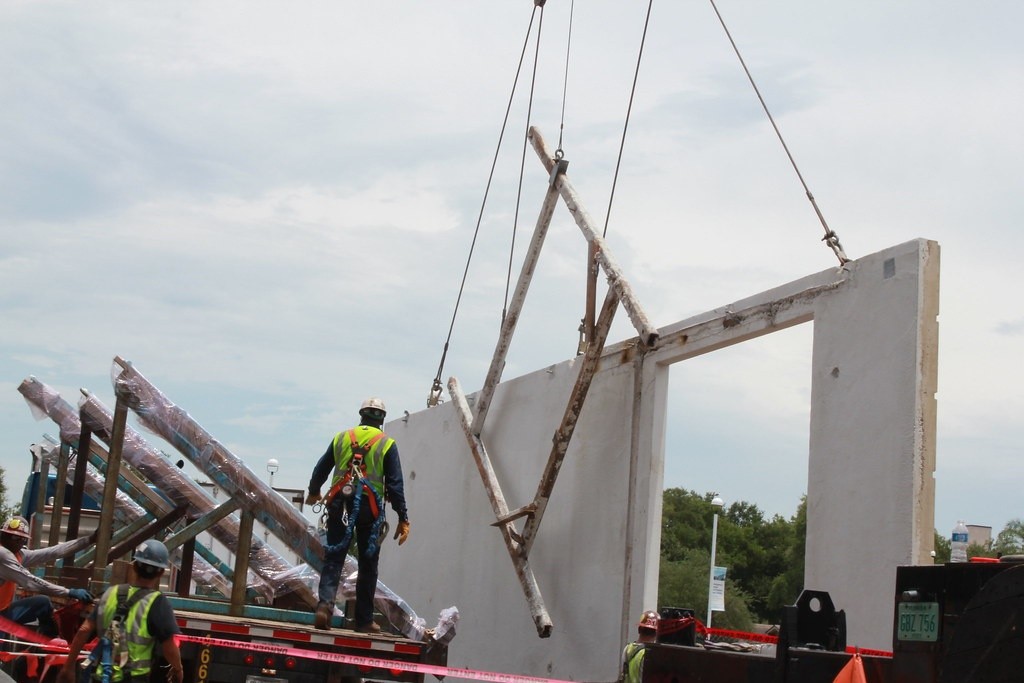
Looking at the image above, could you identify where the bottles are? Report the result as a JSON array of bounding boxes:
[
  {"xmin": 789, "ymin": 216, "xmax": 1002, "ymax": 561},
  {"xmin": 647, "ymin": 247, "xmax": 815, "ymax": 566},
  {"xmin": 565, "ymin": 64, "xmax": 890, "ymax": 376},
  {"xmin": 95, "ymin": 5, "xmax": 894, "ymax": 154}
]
[{"xmin": 949, "ymin": 518, "xmax": 970, "ymax": 563}]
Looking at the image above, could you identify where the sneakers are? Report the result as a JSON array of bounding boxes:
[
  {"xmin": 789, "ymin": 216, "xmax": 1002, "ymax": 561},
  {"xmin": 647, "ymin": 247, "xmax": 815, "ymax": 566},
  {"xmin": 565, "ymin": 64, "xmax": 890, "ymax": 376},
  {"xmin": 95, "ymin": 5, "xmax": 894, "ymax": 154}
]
[
  {"xmin": 353, "ymin": 619, "xmax": 381, "ymax": 634},
  {"xmin": 314, "ymin": 603, "xmax": 332, "ymax": 630}
]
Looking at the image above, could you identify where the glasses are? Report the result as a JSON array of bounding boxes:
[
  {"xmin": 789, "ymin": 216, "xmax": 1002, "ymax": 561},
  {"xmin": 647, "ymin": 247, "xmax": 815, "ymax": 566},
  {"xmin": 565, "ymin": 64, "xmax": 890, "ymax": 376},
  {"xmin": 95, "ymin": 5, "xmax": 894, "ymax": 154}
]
[{"xmin": 11, "ymin": 535, "xmax": 28, "ymax": 544}]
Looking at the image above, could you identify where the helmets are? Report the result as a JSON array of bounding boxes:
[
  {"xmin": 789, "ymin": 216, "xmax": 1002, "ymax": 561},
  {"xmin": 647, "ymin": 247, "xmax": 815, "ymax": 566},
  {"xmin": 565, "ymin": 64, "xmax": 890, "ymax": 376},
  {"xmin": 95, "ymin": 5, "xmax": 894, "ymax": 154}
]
[
  {"xmin": 133, "ymin": 540, "xmax": 169, "ymax": 569},
  {"xmin": 640, "ymin": 610, "xmax": 661, "ymax": 630},
  {"xmin": 0, "ymin": 516, "xmax": 31, "ymax": 539},
  {"xmin": 359, "ymin": 398, "xmax": 386, "ymax": 417}
]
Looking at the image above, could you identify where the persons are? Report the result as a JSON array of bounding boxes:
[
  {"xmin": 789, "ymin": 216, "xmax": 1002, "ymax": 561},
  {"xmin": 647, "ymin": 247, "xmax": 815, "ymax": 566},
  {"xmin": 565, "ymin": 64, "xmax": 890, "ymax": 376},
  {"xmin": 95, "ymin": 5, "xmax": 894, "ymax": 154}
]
[
  {"xmin": 0, "ymin": 515, "xmax": 114, "ymax": 637},
  {"xmin": 305, "ymin": 398, "xmax": 410, "ymax": 632},
  {"xmin": 618, "ymin": 609, "xmax": 662, "ymax": 683},
  {"xmin": 56, "ymin": 539, "xmax": 184, "ymax": 683}
]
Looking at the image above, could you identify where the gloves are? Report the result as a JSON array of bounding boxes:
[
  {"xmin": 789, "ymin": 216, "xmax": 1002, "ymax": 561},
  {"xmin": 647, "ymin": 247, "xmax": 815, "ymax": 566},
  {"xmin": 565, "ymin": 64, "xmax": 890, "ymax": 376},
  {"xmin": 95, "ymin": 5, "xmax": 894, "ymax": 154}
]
[
  {"xmin": 393, "ymin": 522, "xmax": 410, "ymax": 546},
  {"xmin": 305, "ymin": 494, "xmax": 322, "ymax": 507},
  {"xmin": 69, "ymin": 587, "xmax": 96, "ymax": 604}
]
